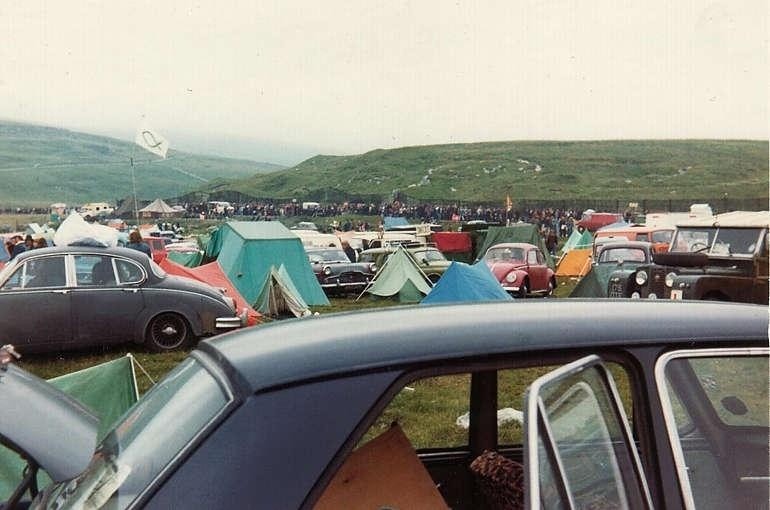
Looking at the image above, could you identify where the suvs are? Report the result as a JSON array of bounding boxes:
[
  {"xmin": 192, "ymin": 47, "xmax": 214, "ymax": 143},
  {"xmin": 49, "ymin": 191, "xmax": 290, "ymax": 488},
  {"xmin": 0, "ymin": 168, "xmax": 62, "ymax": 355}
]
[{"xmin": 607, "ymin": 215, "xmax": 769, "ymax": 305}]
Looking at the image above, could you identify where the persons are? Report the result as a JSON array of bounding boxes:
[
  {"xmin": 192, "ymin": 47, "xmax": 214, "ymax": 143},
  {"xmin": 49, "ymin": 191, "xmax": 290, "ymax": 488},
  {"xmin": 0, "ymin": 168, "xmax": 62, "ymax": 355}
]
[
  {"xmin": 3, "ymin": 234, "xmax": 65, "ymax": 288},
  {"xmin": 85, "ymin": 216, "xmax": 185, "ymax": 286},
  {"xmin": 0, "ymin": 204, "xmax": 83, "ymax": 217},
  {"xmin": 188, "ymin": 198, "xmax": 580, "ymax": 265}
]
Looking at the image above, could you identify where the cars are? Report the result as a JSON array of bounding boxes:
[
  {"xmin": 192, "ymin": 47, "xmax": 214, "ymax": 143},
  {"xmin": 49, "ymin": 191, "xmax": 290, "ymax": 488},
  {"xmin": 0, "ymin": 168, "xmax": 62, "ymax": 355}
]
[
  {"xmin": 1, "ymin": 246, "xmax": 249, "ymax": 353},
  {"xmin": 117, "ymin": 221, "xmax": 185, "ymax": 257},
  {"xmin": 0, "ymin": 298, "xmax": 769, "ymax": 509},
  {"xmin": 307, "ymin": 214, "xmax": 699, "ymax": 299}
]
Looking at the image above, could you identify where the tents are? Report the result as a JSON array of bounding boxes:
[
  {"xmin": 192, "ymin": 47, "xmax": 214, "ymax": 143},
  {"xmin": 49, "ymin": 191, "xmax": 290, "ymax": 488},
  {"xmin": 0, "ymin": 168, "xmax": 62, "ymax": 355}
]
[
  {"xmin": 555, "ymin": 224, "xmax": 599, "ymax": 252},
  {"xmin": 353, "ymin": 239, "xmax": 433, "ymax": 302},
  {"xmin": 421, "ymin": 258, "xmax": 514, "ymax": 304},
  {"xmin": 0, "ymin": 351, "xmax": 144, "ymax": 510},
  {"xmin": 205, "ymin": 220, "xmax": 333, "ymax": 308},
  {"xmin": 551, "ymin": 245, "xmax": 596, "ymax": 278},
  {"xmin": 136, "ymin": 196, "xmax": 185, "ymax": 219},
  {"xmin": 157, "ymin": 256, "xmax": 263, "ymax": 327},
  {"xmin": 110, "ymin": 195, "xmax": 146, "ymax": 220},
  {"xmin": 249, "ymin": 264, "xmax": 315, "ymax": 319},
  {"xmin": 165, "ymin": 251, "xmax": 208, "ymax": 270}
]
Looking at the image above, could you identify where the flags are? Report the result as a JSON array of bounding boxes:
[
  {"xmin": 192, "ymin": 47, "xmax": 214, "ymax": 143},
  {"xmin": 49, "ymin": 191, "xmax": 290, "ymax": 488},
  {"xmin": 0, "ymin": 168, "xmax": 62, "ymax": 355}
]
[
  {"xmin": 506, "ymin": 195, "xmax": 513, "ymax": 211},
  {"xmin": 134, "ymin": 123, "xmax": 169, "ymax": 159}
]
